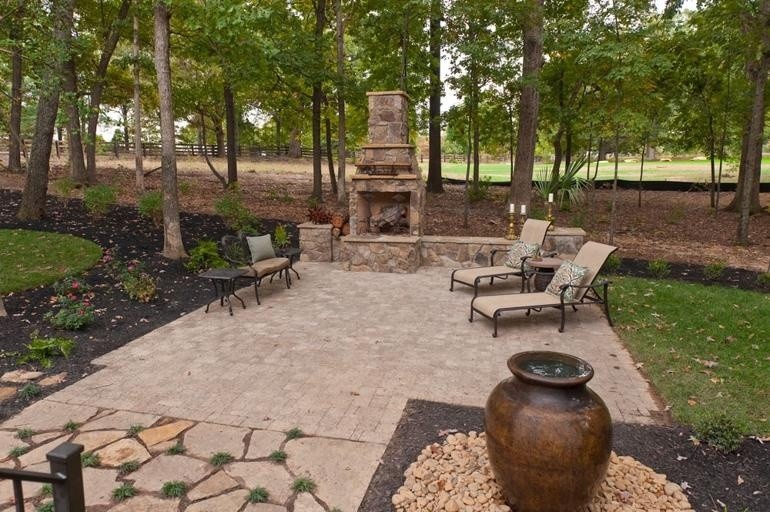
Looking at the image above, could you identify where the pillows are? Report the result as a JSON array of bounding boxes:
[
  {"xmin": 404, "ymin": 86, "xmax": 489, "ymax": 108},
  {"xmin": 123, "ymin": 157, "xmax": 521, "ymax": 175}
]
[{"xmin": 245, "ymin": 233, "xmax": 278, "ymax": 264}]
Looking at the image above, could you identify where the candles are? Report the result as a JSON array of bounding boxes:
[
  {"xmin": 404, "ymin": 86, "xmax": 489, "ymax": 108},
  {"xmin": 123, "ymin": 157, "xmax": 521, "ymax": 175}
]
[{"xmin": 509, "ymin": 192, "xmax": 554, "ymax": 215}]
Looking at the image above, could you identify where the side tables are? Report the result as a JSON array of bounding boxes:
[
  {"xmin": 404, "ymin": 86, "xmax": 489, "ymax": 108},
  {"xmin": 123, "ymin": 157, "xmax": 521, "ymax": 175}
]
[
  {"xmin": 269, "ymin": 246, "xmax": 304, "ymax": 286},
  {"xmin": 202, "ymin": 268, "xmax": 247, "ymax": 316}
]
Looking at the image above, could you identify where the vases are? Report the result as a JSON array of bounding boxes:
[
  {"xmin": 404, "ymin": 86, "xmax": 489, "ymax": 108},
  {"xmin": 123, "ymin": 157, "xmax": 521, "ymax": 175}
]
[{"xmin": 481, "ymin": 351, "xmax": 613, "ymax": 511}]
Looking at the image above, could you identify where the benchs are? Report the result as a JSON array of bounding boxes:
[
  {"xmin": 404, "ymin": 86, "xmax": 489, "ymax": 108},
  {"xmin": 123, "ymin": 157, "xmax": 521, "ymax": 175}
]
[{"xmin": 218, "ymin": 230, "xmax": 291, "ymax": 305}]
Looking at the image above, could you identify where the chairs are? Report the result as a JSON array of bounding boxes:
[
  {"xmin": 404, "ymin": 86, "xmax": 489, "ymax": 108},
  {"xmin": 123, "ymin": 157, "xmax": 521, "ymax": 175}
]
[{"xmin": 450, "ymin": 217, "xmax": 622, "ymax": 338}]
